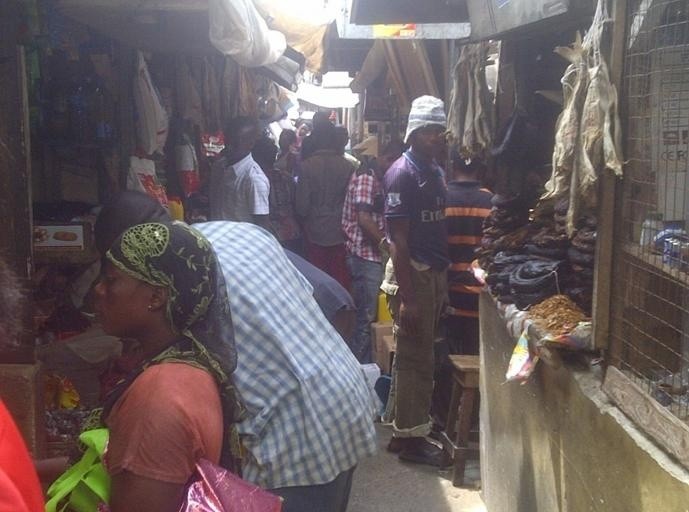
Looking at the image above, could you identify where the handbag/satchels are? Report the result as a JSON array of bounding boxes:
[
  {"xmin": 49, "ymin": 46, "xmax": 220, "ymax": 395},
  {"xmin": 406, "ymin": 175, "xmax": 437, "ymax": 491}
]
[{"xmin": 44, "ymin": 428, "xmax": 111, "ymax": 512}]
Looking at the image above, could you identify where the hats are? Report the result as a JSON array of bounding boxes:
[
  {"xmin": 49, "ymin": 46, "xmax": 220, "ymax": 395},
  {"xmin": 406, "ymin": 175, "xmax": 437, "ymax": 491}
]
[{"xmin": 403, "ymin": 95, "xmax": 446, "ymax": 143}]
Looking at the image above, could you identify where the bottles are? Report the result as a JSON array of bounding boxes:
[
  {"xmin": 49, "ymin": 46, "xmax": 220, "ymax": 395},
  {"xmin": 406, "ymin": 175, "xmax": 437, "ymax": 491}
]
[{"xmin": 639, "ymin": 211, "xmax": 665, "ymax": 249}]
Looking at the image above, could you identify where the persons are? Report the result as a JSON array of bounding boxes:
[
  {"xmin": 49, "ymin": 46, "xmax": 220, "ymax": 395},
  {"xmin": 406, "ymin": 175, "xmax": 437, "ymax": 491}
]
[
  {"xmin": 207, "ymin": 110, "xmax": 391, "ymax": 363},
  {"xmin": 95, "ymin": 192, "xmax": 384, "ymax": 512},
  {"xmin": 94, "ymin": 220, "xmax": 248, "ymax": 512},
  {"xmin": 427, "ymin": 150, "xmax": 495, "ymax": 441},
  {"xmin": 382, "ymin": 95, "xmax": 453, "ymax": 466}
]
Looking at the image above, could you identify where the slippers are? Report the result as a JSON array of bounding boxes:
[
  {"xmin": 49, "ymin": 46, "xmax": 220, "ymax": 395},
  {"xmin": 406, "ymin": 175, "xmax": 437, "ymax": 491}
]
[{"xmin": 389, "ymin": 438, "xmax": 453, "ymax": 467}]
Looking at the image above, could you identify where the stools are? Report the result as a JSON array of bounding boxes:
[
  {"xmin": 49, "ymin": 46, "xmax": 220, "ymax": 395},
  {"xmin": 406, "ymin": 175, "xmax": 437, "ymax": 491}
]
[{"xmin": 435, "ymin": 353, "xmax": 484, "ymax": 487}]
